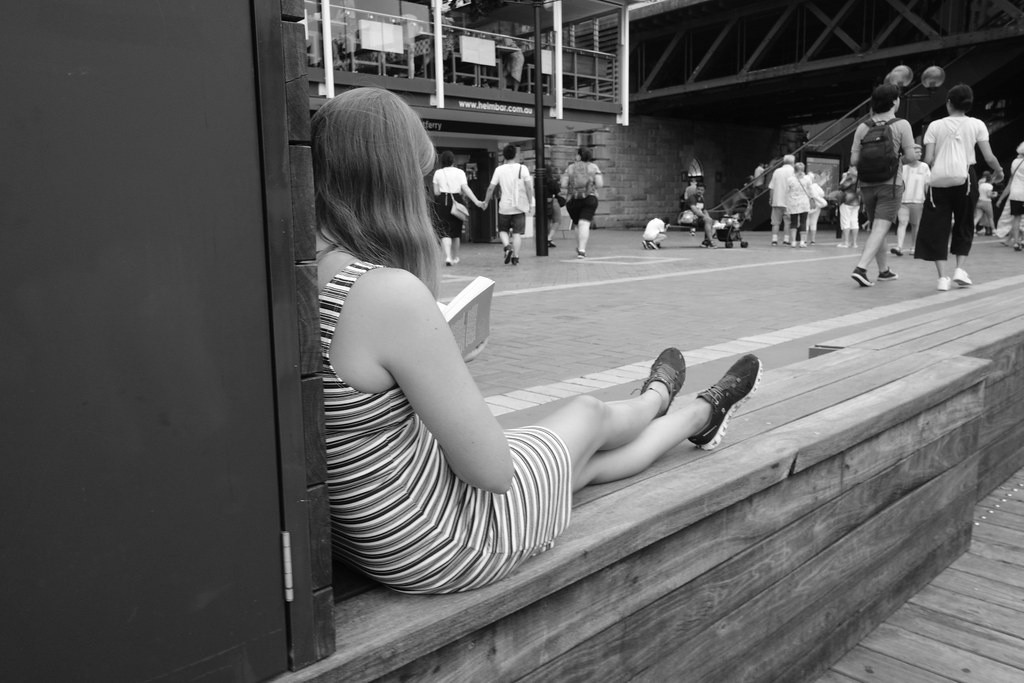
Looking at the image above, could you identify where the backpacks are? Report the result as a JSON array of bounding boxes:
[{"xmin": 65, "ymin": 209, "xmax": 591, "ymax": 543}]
[
  {"xmin": 568, "ymin": 161, "xmax": 593, "ymax": 199},
  {"xmin": 840, "ymin": 172, "xmax": 860, "ymax": 206},
  {"xmin": 855, "ymin": 118, "xmax": 903, "ymax": 198},
  {"xmin": 678, "ymin": 210, "xmax": 694, "ymax": 225},
  {"xmin": 930, "ymin": 117, "xmax": 970, "ymax": 208}
]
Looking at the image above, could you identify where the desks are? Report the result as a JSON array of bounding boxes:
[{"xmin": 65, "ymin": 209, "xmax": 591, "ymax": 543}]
[
  {"xmin": 309, "ymin": 17, "xmax": 348, "ymax": 67},
  {"xmin": 494, "ymin": 45, "xmax": 521, "ymax": 89}
]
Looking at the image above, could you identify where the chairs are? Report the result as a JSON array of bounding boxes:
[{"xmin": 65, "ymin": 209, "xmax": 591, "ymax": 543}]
[{"xmin": 298, "ymin": 9, "xmax": 552, "ymax": 95}]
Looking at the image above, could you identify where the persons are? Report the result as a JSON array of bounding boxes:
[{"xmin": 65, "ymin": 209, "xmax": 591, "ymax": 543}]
[
  {"xmin": 684, "ymin": 182, "xmax": 718, "ymax": 248},
  {"xmin": 560, "ymin": 147, "xmax": 604, "ymax": 258},
  {"xmin": 481, "ymin": 145, "xmax": 533, "ymax": 266},
  {"xmin": 432, "ymin": 151, "xmax": 481, "ymax": 267},
  {"xmin": 743, "ymin": 161, "xmax": 773, "ymax": 197},
  {"xmin": 642, "ymin": 216, "xmax": 671, "ymax": 250},
  {"xmin": 415, "ymin": 7, "xmax": 524, "ymax": 91},
  {"xmin": 312, "ymin": 87, "xmax": 761, "ymax": 596},
  {"xmin": 535, "ymin": 163, "xmax": 562, "ymax": 247},
  {"xmin": 914, "ymin": 83, "xmax": 1005, "ymax": 291},
  {"xmin": 768, "ymin": 142, "xmax": 1024, "ymax": 256},
  {"xmin": 850, "ymin": 83, "xmax": 915, "ymax": 287},
  {"xmin": 684, "ymin": 178, "xmax": 699, "ymax": 236}
]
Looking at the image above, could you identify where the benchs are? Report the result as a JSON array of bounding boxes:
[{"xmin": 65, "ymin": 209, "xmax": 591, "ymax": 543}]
[{"xmin": 269, "ymin": 286, "xmax": 1024, "ymax": 683}]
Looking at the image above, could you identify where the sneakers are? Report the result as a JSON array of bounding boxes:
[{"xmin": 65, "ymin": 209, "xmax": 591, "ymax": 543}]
[
  {"xmin": 687, "ymin": 354, "xmax": 763, "ymax": 450},
  {"xmin": 640, "ymin": 348, "xmax": 686, "ymax": 417}
]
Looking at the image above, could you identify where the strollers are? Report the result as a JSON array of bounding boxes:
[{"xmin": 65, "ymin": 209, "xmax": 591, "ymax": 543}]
[{"xmin": 713, "ymin": 199, "xmax": 753, "ymax": 247}]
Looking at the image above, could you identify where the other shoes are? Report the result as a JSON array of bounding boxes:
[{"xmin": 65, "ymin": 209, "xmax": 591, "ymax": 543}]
[
  {"xmin": 952, "ymin": 268, "xmax": 972, "ymax": 288},
  {"xmin": 850, "ymin": 267, "xmax": 875, "ymax": 287},
  {"xmin": 936, "ymin": 276, "xmax": 953, "ymax": 290},
  {"xmin": 446, "ymin": 257, "xmax": 460, "ymax": 266},
  {"xmin": 878, "ymin": 266, "xmax": 898, "ymax": 281},
  {"xmin": 890, "ymin": 246, "xmax": 904, "ymax": 256},
  {"xmin": 771, "ymin": 242, "xmax": 858, "ymax": 249},
  {"xmin": 974, "ymin": 229, "xmax": 1024, "ymax": 251},
  {"xmin": 908, "ymin": 249, "xmax": 915, "ymax": 255},
  {"xmin": 642, "ymin": 240, "xmax": 661, "ymax": 249},
  {"xmin": 575, "ymin": 248, "xmax": 586, "ymax": 258},
  {"xmin": 700, "ymin": 240, "xmax": 717, "ymax": 248},
  {"xmin": 504, "ymin": 245, "xmax": 519, "ymax": 265},
  {"xmin": 690, "ymin": 232, "xmax": 696, "ymax": 236},
  {"xmin": 547, "ymin": 240, "xmax": 557, "ymax": 247}
]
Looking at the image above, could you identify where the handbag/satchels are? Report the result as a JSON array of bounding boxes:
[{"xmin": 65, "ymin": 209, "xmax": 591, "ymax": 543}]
[
  {"xmin": 810, "ymin": 198, "xmax": 815, "ymax": 209},
  {"xmin": 450, "ymin": 201, "xmax": 469, "ymax": 222},
  {"xmin": 546, "ymin": 200, "xmax": 554, "ymax": 216},
  {"xmin": 513, "ymin": 165, "xmax": 530, "ymax": 213}
]
